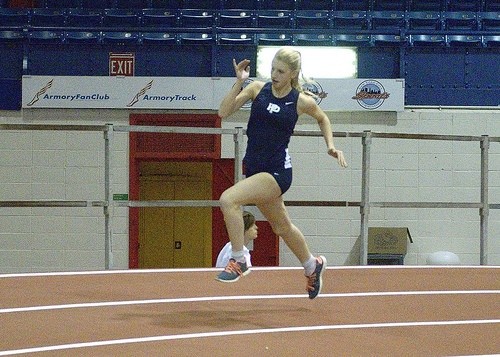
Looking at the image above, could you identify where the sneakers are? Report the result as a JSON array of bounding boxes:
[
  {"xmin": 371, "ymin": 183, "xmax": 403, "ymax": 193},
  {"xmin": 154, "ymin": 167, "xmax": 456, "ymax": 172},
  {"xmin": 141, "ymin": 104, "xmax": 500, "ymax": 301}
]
[
  {"xmin": 217, "ymin": 257, "xmax": 250, "ymax": 283},
  {"xmin": 304, "ymin": 256, "xmax": 326, "ymax": 299}
]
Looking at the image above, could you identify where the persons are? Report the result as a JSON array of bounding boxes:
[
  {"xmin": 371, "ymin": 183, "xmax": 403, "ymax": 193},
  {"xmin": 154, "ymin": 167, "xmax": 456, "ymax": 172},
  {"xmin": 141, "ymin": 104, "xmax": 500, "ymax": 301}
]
[
  {"xmin": 216, "ymin": 212, "xmax": 258, "ymax": 268},
  {"xmin": 215, "ymin": 48, "xmax": 347, "ymax": 300}
]
